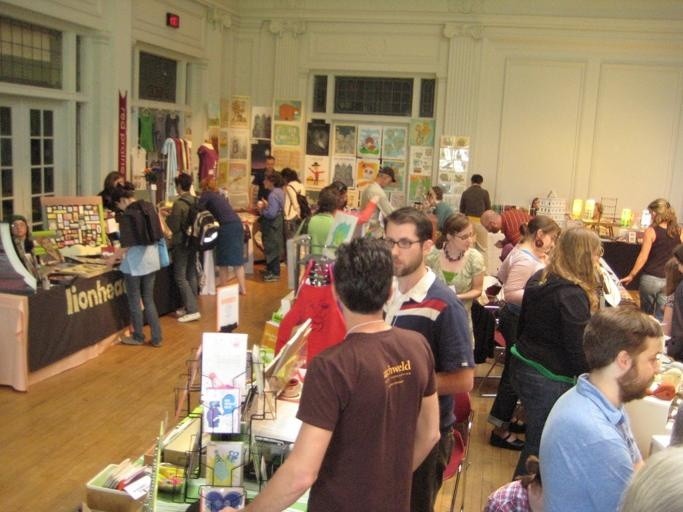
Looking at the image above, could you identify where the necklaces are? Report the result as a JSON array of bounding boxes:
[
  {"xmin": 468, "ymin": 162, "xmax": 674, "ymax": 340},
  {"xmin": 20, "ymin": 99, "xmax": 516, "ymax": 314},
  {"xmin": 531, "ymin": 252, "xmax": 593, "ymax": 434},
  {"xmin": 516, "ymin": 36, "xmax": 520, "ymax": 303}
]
[{"xmin": 340, "ymin": 318, "xmax": 384, "ymax": 338}]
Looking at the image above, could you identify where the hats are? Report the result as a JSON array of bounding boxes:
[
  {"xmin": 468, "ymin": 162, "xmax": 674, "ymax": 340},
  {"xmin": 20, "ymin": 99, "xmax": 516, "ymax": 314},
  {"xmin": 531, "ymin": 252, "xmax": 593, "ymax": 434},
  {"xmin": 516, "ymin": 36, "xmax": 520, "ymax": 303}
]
[{"xmin": 379, "ymin": 167, "xmax": 396, "ymax": 182}]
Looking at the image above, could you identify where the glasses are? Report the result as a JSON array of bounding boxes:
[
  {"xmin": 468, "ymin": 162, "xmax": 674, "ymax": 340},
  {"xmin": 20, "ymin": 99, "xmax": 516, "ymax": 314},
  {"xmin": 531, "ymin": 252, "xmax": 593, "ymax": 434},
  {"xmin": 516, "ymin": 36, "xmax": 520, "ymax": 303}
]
[
  {"xmin": 386, "ymin": 239, "xmax": 420, "ymax": 249},
  {"xmin": 456, "ymin": 233, "xmax": 473, "ymax": 239}
]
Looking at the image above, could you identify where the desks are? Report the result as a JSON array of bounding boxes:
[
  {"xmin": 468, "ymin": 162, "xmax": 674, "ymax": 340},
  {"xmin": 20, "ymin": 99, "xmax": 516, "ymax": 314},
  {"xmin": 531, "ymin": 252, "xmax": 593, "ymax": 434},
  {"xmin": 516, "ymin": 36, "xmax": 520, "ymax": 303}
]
[
  {"xmin": 598, "ymin": 234, "xmax": 641, "ymax": 290},
  {"xmin": 0, "ymin": 238, "xmax": 201, "ymax": 394},
  {"xmin": 130, "ymin": 366, "xmax": 310, "ymax": 511}
]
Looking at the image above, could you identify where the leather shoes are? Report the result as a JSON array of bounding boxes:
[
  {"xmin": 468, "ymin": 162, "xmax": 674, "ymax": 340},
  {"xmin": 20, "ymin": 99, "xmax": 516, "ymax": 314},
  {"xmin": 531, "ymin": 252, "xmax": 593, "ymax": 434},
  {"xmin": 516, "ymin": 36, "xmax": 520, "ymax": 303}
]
[{"xmin": 491, "ymin": 417, "xmax": 525, "ymax": 450}]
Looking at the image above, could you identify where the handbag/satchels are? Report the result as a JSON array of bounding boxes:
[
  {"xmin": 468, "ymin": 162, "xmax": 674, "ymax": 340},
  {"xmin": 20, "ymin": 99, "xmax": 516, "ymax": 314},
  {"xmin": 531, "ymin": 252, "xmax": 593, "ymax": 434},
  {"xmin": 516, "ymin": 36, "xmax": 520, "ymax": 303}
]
[{"xmin": 297, "ymin": 193, "xmax": 311, "ymax": 217}]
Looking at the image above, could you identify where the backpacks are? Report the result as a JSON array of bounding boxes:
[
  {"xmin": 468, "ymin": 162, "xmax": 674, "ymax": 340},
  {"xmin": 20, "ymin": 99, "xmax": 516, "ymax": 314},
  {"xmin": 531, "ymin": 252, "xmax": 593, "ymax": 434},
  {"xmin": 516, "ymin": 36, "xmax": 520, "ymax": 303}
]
[{"xmin": 184, "ymin": 200, "xmax": 220, "ymax": 252}]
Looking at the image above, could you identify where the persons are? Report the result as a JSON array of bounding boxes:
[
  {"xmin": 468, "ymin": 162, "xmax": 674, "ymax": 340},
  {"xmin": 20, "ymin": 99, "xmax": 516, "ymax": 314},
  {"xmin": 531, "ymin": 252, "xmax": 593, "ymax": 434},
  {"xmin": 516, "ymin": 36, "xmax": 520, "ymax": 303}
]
[
  {"xmin": 199, "ymin": 140, "xmax": 220, "ymax": 181},
  {"xmin": 481, "ymin": 456, "xmax": 542, "ymax": 512},
  {"xmin": 98, "ymin": 160, "xmax": 247, "ymax": 347},
  {"xmin": 255, "ymin": 169, "xmax": 308, "ymax": 285},
  {"xmin": 616, "ymin": 445, "xmax": 681, "ymax": 512},
  {"xmin": 306, "ymin": 167, "xmax": 682, "ymax": 362},
  {"xmin": 488, "ymin": 216, "xmax": 561, "ymax": 452},
  {"xmin": 382, "ymin": 206, "xmax": 473, "ymax": 512},
  {"xmin": 538, "ymin": 303, "xmax": 663, "ymax": 512},
  {"xmin": 506, "ymin": 226, "xmax": 605, "ymax": 481},
  {"xmin": 6, "ymin": 214, "xmax": 35, "ymax": 261},
  {"xmin": 218, "ymin": 239, "xmax": 443, "ymax": 512}
]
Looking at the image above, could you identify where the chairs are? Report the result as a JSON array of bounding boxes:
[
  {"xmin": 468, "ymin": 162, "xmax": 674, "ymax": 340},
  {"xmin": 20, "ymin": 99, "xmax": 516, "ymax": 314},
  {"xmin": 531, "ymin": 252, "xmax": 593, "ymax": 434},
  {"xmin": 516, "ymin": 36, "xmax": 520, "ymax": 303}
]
[
  {"xmin": 441, "ymin": 391, "xmax": 474, "ymax": 512},
  {"xmin": 473, "ymin": 305, "xmax": 507, "ymax": 398}
]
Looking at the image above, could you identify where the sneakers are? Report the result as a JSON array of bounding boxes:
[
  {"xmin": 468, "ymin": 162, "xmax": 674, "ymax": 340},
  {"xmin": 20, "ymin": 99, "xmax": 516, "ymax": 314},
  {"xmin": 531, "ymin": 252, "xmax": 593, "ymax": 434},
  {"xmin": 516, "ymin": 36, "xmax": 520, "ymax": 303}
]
[
  {"xmin": 122, "ymin": 337, "xmax": 143, "ymax": 345},
  {"xmin": 264, "ymin": 271, "xmax": 279, "ymax": 281},
  {"xmin": 176, "ymin": 309, "xmax": 201, "ymax": 322}
]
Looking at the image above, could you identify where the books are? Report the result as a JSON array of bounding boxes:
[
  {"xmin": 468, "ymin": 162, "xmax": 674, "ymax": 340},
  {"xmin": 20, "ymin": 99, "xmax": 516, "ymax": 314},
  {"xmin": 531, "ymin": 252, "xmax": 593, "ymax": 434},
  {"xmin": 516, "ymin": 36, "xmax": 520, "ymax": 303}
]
[{"xmin": 98, "ymin": 318, "xmax": 310, "ymax": 511}]
